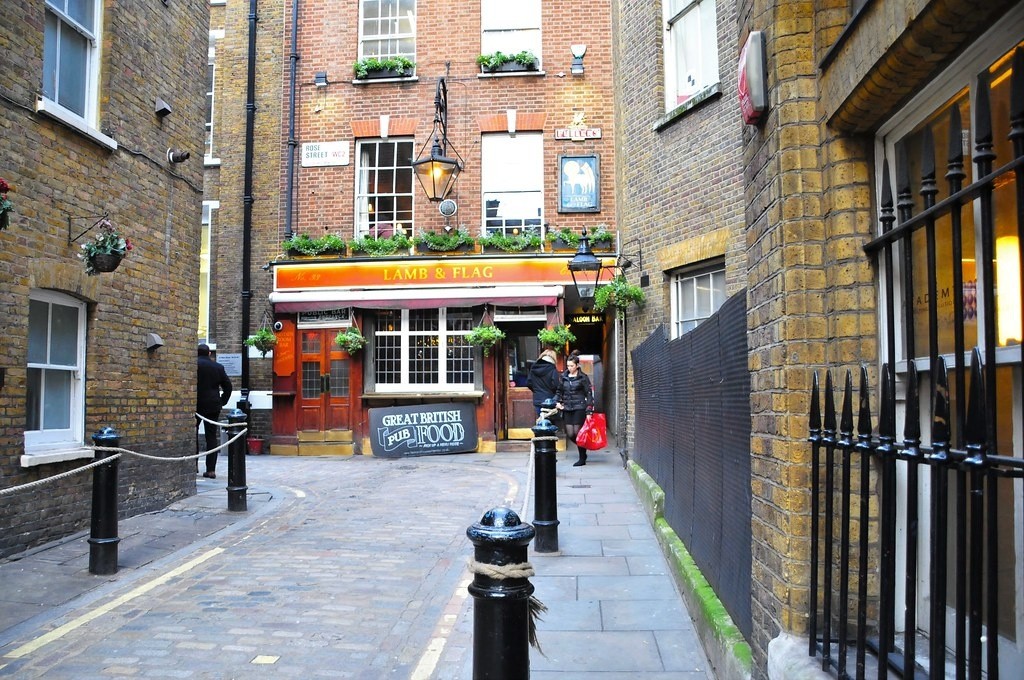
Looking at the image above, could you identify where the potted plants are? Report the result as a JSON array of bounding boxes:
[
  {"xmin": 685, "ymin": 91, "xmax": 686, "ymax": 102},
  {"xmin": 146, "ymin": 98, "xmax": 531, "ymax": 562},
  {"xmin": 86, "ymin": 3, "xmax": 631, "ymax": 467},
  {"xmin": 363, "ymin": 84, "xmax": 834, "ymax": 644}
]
[
  {"xmin": 353, "ymin": 55, "xmax": 416, "ymax": 78},
  {"xmin": 593, "ymin": 278, "xmax": 649, "ymax": 313},
  {"xmin": 465, "ymin": 325, "xmax": 506, "ymax": 358},
  {"xmin": 547, "ymin": 227, "xmax": 615, "ymax": 250},
  {"xmin": 537, "ymin": 326, "xmax": 577, "ymax": 353},
  {"xmin": 335, "ymin": 328, "xmax": 369, "ymax": 356},
  {"xmin": 350, "ymin": 232, "xmax": 413, "ymax": 256},
  {"xmin": 284, "ymin": 229, "xmax": 347, "ymax": 255},
  {"xmin": 482, "ymin": 229, "xmax": 546, "ymax": 253},
  {"xmin": 411, "ymin": 225, "xmax": 474, "ymax": 253},
  {"xmin": 477, "ymin": 49, "xmax": 537, "ymax": 73},
  {"xmin": 243, "ymin": 327, "xmax": 280, "ymax": 358}
]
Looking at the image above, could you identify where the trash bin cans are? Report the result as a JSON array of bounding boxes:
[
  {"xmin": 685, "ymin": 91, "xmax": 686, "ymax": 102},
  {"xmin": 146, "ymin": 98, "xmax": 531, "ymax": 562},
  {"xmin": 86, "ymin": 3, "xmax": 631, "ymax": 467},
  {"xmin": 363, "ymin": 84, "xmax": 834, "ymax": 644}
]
[{"xmin": 220, "ymin": 420, "xmax": 230, "ymax": 455}]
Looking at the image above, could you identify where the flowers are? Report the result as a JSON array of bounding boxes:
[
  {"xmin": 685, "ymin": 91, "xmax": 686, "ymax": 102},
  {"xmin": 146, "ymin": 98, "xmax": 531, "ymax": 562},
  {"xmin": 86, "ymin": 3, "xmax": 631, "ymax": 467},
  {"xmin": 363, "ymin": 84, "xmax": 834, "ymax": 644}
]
[
  {"xmin": 0, "ymin": 177, "xmax": 16, "ymax": 228},
  {"xmin": 76, "ymin": 218, "xmax": 132, "ymax": 277}
]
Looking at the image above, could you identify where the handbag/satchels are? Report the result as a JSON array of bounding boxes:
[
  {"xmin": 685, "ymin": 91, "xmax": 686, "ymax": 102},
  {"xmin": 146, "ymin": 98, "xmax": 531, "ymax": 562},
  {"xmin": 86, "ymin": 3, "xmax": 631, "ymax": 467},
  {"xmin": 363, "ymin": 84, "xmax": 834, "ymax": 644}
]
[{"xmin": 575, "ymin": 406, "xmax": 608, "ymax": 450}]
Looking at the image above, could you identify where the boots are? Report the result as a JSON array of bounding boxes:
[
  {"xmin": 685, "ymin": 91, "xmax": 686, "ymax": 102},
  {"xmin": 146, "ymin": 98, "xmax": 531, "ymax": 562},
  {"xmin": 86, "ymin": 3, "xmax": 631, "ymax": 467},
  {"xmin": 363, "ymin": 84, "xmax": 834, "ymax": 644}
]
[
  {"xmin": 569, "ymin": 435, "xmax": 587, "ymax": 460},
  {"xmin": 573, "ymin": 446, "xmax": 586, "ymax": 466}
]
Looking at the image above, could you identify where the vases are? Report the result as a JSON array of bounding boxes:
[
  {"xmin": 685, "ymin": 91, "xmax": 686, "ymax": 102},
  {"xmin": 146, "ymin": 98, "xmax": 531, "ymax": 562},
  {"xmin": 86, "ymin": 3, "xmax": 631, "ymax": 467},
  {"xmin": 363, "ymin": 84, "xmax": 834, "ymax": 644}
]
[{"xmin": 89, "ymin": 253, "xmax": 127, "ymax": 271}]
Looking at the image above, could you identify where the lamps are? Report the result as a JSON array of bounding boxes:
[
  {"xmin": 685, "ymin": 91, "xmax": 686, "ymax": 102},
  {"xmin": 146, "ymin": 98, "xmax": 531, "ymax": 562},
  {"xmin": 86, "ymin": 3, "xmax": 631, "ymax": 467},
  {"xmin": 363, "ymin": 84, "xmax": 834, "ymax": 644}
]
[
  {"xmin": 567, "ymin": 224, "xmax": 649, "ymax": 313},
  {"xmin": 155, "ymin": 97, "xmax": 171, "ymax": 116},
  {"xmin": 570, "ymin": 59, "xmax": 585, "ymax": 75},
  {"xmin": 412, "ymin": 78, "xmax": 462, "ymax": 203},
  {"xmin": 314, "ymin": 72, "xmax": 327, "ymax": 87},
  {"xmin": 147, "ymin": 333, "xmax": 164, "ymax": 350}
]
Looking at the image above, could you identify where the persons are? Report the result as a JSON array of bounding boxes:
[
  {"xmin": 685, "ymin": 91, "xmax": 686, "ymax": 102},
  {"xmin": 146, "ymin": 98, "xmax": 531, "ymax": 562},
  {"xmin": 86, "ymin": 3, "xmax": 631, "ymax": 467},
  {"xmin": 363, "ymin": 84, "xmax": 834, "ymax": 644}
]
[
  {"xmin": 368, "ymin": 207, "xmax": 393, "ymax": 239},
  {"xmin": 526, "ymin": 349, "xmax": 560, "ymax": 423},
  {"xmin": 552, "ymin": 350, "xmax": 593, "ymax": 467},
  {"xmin": 195, "ymin": 344, "xmax": 233, "ymax": 477}
]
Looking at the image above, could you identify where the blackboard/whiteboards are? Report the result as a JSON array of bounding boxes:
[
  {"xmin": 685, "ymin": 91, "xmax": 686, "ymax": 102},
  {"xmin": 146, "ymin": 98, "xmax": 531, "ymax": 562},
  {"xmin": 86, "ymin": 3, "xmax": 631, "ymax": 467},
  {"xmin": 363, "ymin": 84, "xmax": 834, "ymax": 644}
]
[
  {"xmin": 368, "ymin": 402, "xmax": 479, "ymax": 457},
  {"xmin": 299, "ymin": 308, "xmax": 349, "ymax": 324}
]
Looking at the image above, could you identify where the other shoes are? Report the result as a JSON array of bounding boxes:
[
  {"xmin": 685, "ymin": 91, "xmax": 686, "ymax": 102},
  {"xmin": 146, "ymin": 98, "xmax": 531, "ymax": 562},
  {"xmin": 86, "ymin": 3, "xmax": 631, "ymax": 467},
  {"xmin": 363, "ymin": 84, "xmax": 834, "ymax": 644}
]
[{"xmin": 203, "ymin": 471, "xmax": 216, "ymax": 478}]
